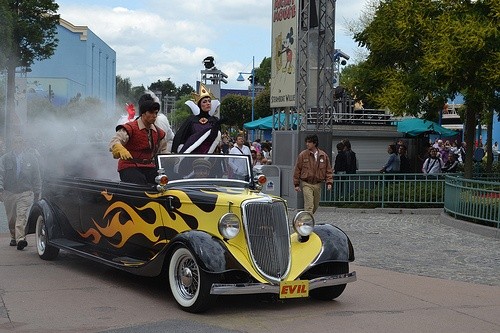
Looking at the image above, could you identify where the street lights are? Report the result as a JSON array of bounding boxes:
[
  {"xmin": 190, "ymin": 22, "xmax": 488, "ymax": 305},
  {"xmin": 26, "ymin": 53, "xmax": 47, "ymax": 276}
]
[{"xmin": 234, "ymin": 55, "xmax": 256, "ymax": 143}]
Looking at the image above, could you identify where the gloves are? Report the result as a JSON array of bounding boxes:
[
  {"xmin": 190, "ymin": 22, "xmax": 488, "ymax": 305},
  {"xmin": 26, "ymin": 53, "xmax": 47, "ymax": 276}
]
[{"xmin": 112, "ymin": 143, "xmax": 133, "ymax": 161}]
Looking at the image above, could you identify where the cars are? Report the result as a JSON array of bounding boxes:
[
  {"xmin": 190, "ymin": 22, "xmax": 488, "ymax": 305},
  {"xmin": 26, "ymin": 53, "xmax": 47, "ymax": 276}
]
[{"xmin": 21, "ymin": 153, "xmax": 358, "ymax": 315}]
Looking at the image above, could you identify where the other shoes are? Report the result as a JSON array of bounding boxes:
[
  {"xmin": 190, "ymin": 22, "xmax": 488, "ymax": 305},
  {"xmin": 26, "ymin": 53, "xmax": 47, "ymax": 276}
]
[
  {"xmin": 10, "ymin": 240, "xmax": 16, "ymax": 246},
  {"xmin": 17, "ymin": 240, "xmax": 28, "ymax": 250}
]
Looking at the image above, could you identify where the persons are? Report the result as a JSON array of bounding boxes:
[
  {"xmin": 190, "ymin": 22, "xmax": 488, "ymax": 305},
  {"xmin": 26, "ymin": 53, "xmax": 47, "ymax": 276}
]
[
  {"xmin": 379, "ymin": 140, "xmax": 408, "ymax": 184},
  {"xmin": 192, "ymin": 158, "xmax": 213, "ymax": 178},
  {"xmin": 293, "ymin": 134, "xmax": 333, "ymax": 227},
  {"xmin": 170, "ymin": 82, "xmax": 224, "ymax": 178},
  {"xmin": 116, "ymin": 89, "xmax": 176, "ymax": 154},
  {"xmin": 112, "ymin": 101, "xmax": 163, "ymax": 186},
  {"xmin": 334, "ymin": 137, "xmax": 359, "ymax": 200},
  {"xmin": 0, "ymin": 132, "xmax": 44, "ymax": 249},
  {"xmin": 219, "ymin": 134, "xmax": 274, "ymax": 178},
  {"xmin": 422, "ymin": 138, "xmax": 500, "ymax": 178}
]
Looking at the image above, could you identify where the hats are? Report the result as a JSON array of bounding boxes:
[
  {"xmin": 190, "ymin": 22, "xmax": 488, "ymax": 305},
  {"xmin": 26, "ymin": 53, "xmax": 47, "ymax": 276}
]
[
  {"xmin": 192, "ymin": 159, "xmax": 212, "ymax": 170},
  {"xmin": 138, "ymin": 94, "xmax": 160, "ymax": 116}
]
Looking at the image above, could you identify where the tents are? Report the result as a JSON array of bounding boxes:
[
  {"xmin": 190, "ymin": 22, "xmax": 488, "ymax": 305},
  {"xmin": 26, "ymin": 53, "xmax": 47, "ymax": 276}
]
[
  {"xmin": 243, "ymin": 112, "xmax": 308, "ymax": 140},
  {"xmin": 392, "ymin": 116, "xmax": 459, "ymax": 141}
]
